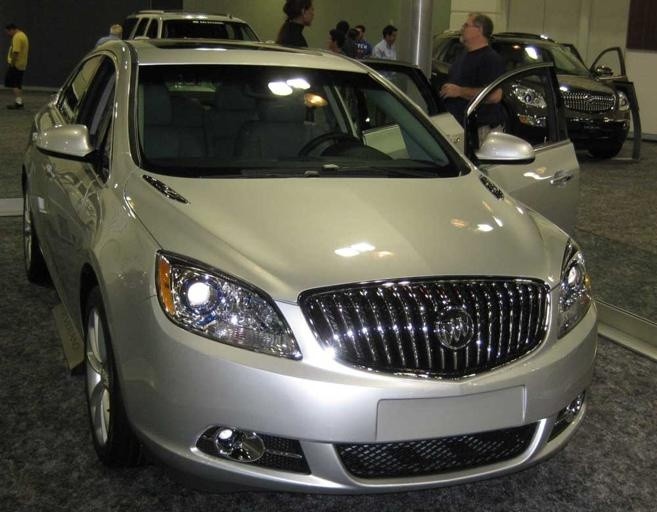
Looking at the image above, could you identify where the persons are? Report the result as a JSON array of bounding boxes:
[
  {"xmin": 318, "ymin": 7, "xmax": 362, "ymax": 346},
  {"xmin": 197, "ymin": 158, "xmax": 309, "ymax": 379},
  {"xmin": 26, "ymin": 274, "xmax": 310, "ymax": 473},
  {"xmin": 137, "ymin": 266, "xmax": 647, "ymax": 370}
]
[
  {"xmin": 437, "ymin": 13, "xmax": 505, "ymax": 150},
  {"xmin": 3, "ymin": 22, "xmax": 30, "ymax": 110},
  {"xmin": 326, "ymin": 20, "xmax": 398, "ymax": 85},
  {"xmin": 276, "ymin": 0, "xmax": 314, "ymax": 48},
  {"xmin": 95, "ymin": 24, "xmax": 123, "ymax": 47}
]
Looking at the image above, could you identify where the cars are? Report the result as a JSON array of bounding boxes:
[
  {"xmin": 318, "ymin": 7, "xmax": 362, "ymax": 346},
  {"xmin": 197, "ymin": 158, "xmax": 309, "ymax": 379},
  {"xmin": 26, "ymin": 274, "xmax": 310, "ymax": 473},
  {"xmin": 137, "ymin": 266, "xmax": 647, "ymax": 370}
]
[{"xmin": 10, "ymin": 36, "xmax": 603, "ymax": 500}]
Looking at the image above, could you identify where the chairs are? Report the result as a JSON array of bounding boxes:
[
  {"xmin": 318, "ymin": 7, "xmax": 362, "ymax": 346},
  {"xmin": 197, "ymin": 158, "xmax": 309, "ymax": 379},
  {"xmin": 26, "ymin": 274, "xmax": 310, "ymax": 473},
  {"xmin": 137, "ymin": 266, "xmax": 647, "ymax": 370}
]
[
  {"xmin": 234, "ymin": 87, "xmax": 331, "ymax": 162},
  {"xmin": 206, "ymin": 80, "xmax": 262, "ymax": 159},
  {"xmin": 141, "ymin": 82, "xmax": 184, "ymax": 166}
]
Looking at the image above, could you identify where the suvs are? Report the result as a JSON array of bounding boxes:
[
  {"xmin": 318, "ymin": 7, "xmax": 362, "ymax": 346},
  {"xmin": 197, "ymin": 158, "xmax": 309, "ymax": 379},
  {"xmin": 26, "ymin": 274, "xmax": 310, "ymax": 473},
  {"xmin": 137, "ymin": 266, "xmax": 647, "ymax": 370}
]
[
  {"xmin": 426, "ymin": 22, "xmax": 638, "ymax": 160},
  {"xmin": 118, "ymin": 5, "xmax": 273, "ymax": 45}
]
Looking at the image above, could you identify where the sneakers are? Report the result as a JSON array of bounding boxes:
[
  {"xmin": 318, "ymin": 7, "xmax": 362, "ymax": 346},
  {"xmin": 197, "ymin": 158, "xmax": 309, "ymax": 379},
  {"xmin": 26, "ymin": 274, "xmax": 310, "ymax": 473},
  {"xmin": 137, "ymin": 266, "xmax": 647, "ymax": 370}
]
[{"xmin": 6, "ymin": 102, "xmax": 25, "ymax": 110}]
